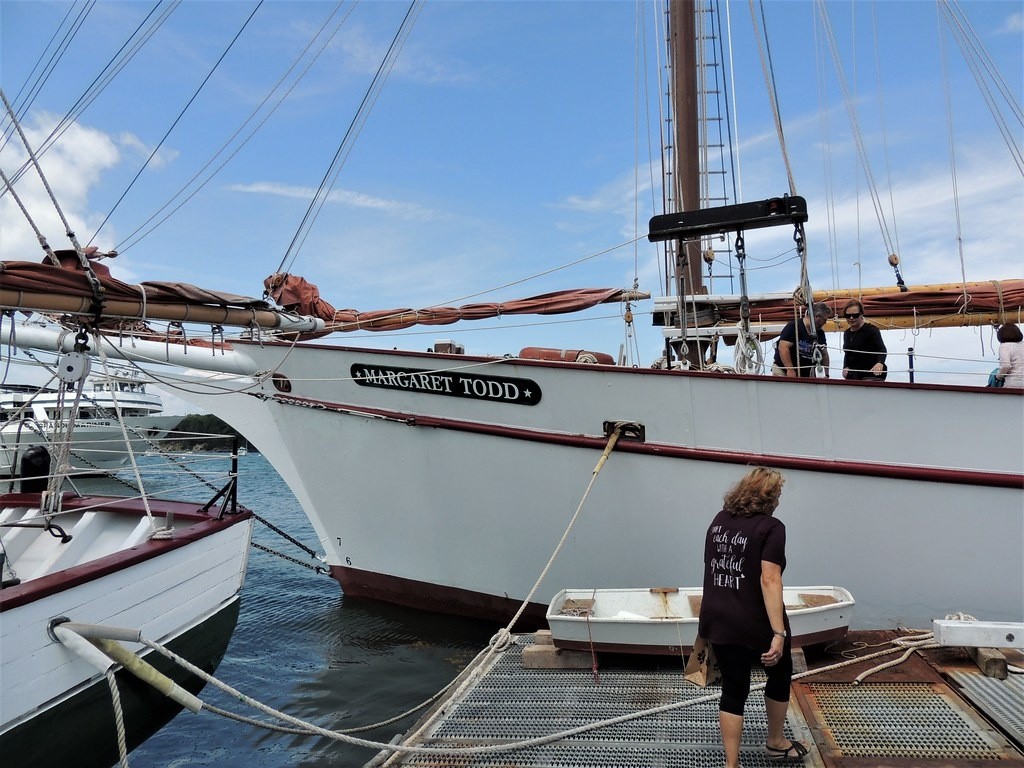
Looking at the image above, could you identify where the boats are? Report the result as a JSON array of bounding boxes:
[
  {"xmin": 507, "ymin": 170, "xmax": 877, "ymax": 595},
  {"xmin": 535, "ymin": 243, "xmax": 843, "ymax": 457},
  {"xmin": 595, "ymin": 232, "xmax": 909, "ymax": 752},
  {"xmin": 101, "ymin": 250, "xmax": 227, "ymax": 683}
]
[
  {"xmin": 547, "ymin": 586, "xmax": 855, "ymax": 657},
  {"xmin": 1, "ymin": 360, "xmax": 189, "ymax": 480},
  {"xmin": 238, "ymin": 446, "xmax": 247, "ymax": 456}
]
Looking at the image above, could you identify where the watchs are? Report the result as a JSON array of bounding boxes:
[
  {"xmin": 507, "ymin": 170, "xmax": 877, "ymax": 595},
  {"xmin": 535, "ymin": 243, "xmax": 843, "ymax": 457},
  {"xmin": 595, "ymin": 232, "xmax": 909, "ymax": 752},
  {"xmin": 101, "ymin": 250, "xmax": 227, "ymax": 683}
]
[{"xmin": 774, "ymin": 631, "xmax": 786, "ymax": 637}]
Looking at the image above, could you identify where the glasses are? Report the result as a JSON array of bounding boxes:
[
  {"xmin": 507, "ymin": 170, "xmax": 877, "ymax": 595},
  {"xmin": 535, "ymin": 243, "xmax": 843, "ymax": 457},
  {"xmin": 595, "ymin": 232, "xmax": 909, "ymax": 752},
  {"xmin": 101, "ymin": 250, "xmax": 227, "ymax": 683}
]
[
  {"xmin": 844, "ymin": 313, "xmax": 861, "ymax": 319},
  {"xmin": 817, "ymin": 315, "xmax": 827, "ymax": 324}
]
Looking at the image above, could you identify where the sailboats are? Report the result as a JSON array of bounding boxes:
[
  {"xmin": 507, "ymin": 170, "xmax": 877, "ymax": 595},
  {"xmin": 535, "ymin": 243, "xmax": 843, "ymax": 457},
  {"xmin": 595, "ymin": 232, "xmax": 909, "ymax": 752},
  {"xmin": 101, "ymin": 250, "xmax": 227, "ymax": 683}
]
[
  {"xmin": 0, "ymin": 0, "xmax": 1024, "ymax": 631},
  {"xmin": 0, "ymin": 89, "xmax": 652, "ymax": 768}
]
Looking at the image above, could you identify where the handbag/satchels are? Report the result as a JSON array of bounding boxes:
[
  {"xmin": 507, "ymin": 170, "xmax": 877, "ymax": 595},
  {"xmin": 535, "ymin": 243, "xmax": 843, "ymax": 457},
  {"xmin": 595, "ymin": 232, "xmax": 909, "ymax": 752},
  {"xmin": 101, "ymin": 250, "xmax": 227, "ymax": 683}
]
[{"xmin": 683, "ymin": 633, "xmax": 722, "ymax": 689}]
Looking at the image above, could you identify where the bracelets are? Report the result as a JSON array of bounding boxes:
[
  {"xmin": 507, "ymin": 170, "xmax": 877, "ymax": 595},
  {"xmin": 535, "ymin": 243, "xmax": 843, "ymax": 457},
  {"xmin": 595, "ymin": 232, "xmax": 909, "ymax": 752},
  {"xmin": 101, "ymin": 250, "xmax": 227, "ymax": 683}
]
[{"xmin": 825, "ymin": 374, "xmax": 829, "ymax": 378}]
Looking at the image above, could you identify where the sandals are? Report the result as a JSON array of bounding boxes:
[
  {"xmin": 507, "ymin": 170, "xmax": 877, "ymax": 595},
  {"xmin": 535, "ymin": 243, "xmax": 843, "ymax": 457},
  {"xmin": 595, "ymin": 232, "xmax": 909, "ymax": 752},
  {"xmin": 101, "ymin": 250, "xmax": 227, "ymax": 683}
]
[{"xmin": 766, "ymin": 739, "xmax": 811, "ymax": 761}]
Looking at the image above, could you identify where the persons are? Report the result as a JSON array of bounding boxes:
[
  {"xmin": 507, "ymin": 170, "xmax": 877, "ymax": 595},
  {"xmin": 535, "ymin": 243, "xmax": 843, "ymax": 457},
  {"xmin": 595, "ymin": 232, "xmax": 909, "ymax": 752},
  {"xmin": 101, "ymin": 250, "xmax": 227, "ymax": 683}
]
[
  {"xmin": 842, "ymin": 299, "xmax": 887, "ymax": 381},
  {"xmin": 995, "ymin": 322, "xmax": 1024, "ymax": 389},
  {"xmin": 771, "ymin": 303, "xmax": 831, "ymax": 379},
  {"xmin": 698, "ymin": 467, "xmax": 812, "ymax": 768}
]
